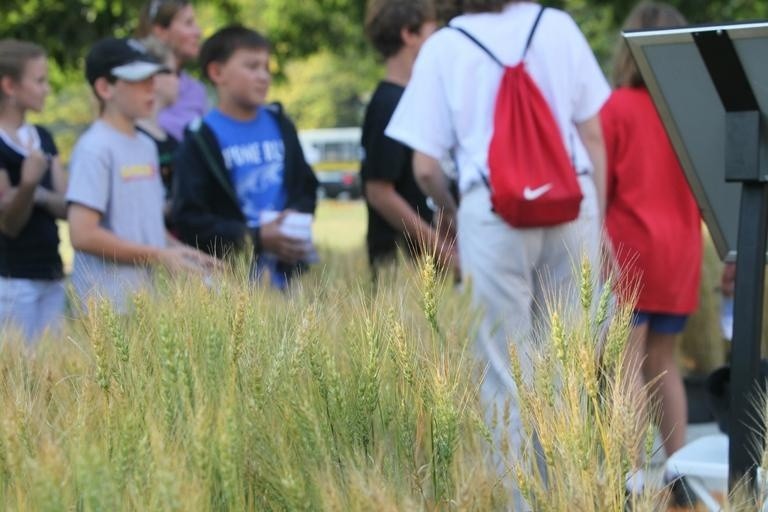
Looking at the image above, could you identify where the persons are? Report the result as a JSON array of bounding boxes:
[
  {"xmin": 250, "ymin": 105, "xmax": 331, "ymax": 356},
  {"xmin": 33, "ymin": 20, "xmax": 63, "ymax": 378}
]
[
  {"xmin": 131, "ymin": 0, "xmax": 212, "ymax": 146},
  {"xmin": 384, "ymin": 2, "xmax": 610, "ymax": 511},
  {"xmin": 164, "ymin": 22, "xmax": 321, "ymax": 301},
  {"xmin": 599, "ymin": 3, "xmax": 737, "ymax": 497},
  {"xmin": 131, "ymin": 35, "xmax": 182, "ymax": 202},
  {"xmin": 0, "ymin": 41, "xmax": 69, "ymax": 346},
  {"xmin": 357, "ymin": 0, "xmax": 459, "ymax": 296},
  {"xmin": 56, "ymin": 31, "xmax": 232, "ymax": 318}
]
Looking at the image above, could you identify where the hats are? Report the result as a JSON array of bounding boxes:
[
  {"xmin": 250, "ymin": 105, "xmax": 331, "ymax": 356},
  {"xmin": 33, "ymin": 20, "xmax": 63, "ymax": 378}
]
[{"xmin": 85, "ymin": 38, "xmax": 172, "ymax": 83}]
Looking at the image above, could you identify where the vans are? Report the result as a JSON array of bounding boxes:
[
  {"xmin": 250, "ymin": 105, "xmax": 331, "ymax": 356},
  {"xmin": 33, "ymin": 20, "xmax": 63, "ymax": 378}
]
[{"xmin": 298, "ymin": 126, "xmax": 365, "ymax": 201}]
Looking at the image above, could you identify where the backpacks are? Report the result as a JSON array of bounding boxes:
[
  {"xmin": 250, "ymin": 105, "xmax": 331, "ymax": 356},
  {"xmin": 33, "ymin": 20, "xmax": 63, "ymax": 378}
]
[{"xmin": 446, "ymin": 5, "xmax": 583, "ymax": 227}]
